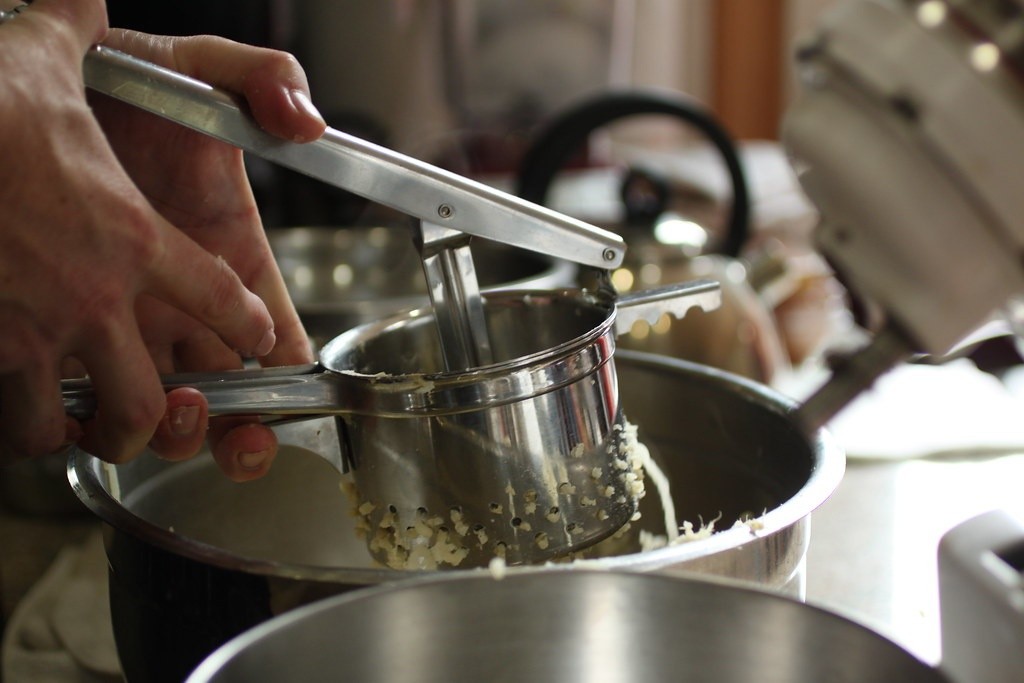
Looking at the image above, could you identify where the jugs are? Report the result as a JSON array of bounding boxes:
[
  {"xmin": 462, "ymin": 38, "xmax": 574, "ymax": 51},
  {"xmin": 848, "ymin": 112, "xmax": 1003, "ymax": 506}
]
[{"xmin": 517, "ymin": 91, "xmax": 807, "ymax": 397}]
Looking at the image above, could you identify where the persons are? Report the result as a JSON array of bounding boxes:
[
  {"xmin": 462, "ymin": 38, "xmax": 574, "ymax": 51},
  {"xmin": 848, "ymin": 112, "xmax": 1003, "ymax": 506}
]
[{"xmin": 0, "ymin": 0, "xmax": 327, "ymax": 482}]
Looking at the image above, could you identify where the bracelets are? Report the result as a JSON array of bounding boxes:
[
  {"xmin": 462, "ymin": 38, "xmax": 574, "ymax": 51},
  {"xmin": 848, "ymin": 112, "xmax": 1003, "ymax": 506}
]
[{"xmin": 3, "ymin": 4, "xmax": 27, "ymax": 23}]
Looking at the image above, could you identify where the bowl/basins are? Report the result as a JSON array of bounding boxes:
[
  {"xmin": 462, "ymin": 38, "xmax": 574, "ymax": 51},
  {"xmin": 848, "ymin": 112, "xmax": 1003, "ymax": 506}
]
[
  {"xmin": 181, "ymin": 567, "xmax": 945, "ymax": 682},
  {"xmin": 69, "ymin": 348, "xmax": 845, "ymax": 683}
]
